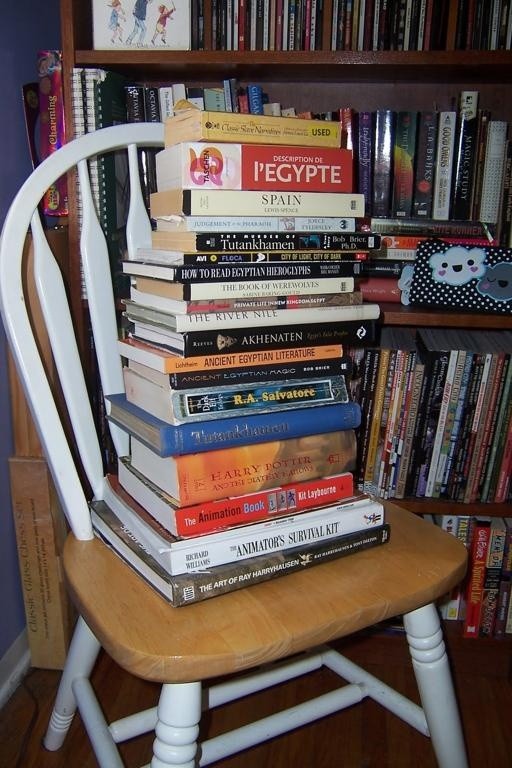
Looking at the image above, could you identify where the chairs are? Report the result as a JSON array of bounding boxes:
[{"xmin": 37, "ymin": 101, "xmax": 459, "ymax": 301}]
[{"xmin": 0, "ymin": 121, "xmax": 479, "ymax": 766}]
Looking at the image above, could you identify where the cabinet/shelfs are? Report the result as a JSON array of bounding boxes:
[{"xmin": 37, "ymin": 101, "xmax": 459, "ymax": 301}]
[{"xmin": 57, "ymin": 0, "xmax": 511, "ymax": 651}]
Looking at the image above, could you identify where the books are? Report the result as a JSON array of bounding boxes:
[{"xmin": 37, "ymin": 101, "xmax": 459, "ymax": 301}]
[
  {"xmin": 90, "ymin": 322, "xmax": 511, "ymax": 608},
  {"xmin": 191, "ymin": 0, "xmax": 512, "ymax": 51},
  {"xmin": 437, "ymin": 515, "xmax": 512, "ymax": 638},
  {"xmin": 70, "ymin": 68, "xmax": 512, "ymax": 333}
]
